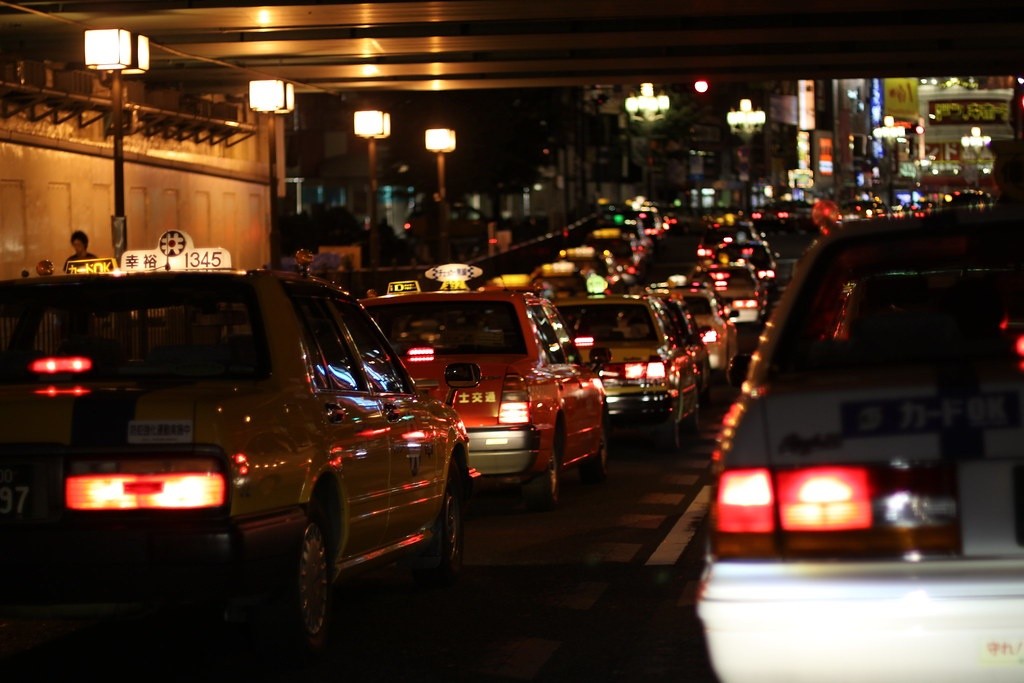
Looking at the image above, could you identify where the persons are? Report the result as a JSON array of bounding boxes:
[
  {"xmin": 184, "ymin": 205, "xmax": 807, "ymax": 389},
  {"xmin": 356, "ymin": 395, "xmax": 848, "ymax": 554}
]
[{"xmin": 62, "ymin": 230, "xmax": 99, "ymax": 270}]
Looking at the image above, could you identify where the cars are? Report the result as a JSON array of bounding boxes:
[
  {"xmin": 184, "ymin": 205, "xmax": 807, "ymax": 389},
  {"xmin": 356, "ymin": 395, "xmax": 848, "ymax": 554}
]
[
  {"xmin": 0, "ymin": 227, "xmax": 474, "ymax": 659},
  {"xmin": 498, "ymin": 196, "xmax": 825, "ymax": 382},
  {"xmin": 351, "ymin": 279, "xmax": 611, "ymax": 513},
  {"xmin": 690, "ymin": 205, "xmax": 1024, "ymax": 683},
  {"xmin": 555, "ymin": 291, "xmax": 700, "ymax": 455}
]
